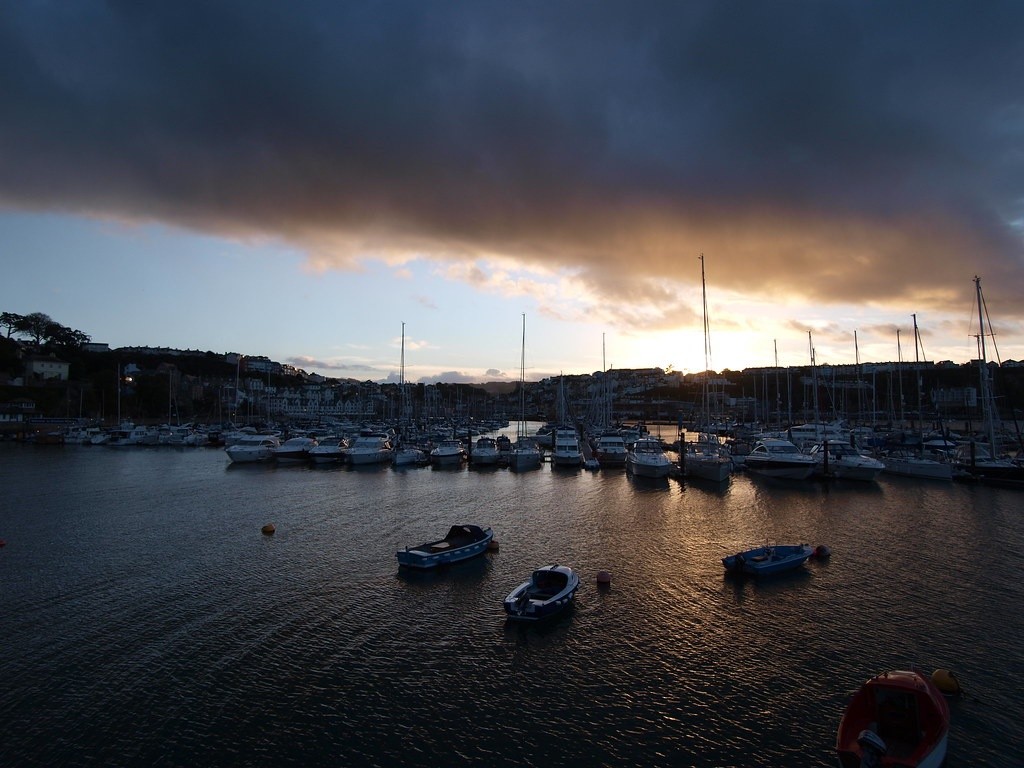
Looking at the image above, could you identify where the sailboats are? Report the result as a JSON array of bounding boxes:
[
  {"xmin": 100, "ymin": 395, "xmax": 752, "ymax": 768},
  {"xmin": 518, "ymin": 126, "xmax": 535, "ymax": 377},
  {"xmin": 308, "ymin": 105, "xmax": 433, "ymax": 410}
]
[{"xmin": 0, "ymin": 254, "xmax": 1024, "ymax": 478}]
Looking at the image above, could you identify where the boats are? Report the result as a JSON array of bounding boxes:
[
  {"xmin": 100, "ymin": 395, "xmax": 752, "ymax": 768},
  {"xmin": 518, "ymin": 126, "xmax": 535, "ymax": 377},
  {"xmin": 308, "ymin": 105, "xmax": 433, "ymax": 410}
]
[
  {"xmin": 504, "ymin": 563, "xmax": 580, "ymax": 617},
  {"xmin": 836, "ymin": 668, "xmax": 963, "ymax": 768},
  {"xmin": 395, "ymin": 525, "xmax": 495, "ymax": 571},
  {"xmin": 722, "ymin": 541, "xmax": 832, "ymax": 586}
]
